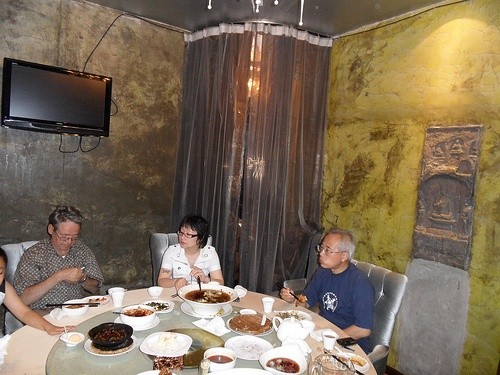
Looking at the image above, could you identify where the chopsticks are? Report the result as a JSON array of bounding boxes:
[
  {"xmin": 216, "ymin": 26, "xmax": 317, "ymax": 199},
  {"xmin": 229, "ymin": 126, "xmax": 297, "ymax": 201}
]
[
  {"xmin": 45, "ymin": 303, "xmax": 100, "ymax": 308},
  {"xmin": 323, "ymin": 349, "xmax": 364, "ymax": 375},
  {"xmin": 274, "ymin": 282, "xmax": 303, "ymax": 303}
]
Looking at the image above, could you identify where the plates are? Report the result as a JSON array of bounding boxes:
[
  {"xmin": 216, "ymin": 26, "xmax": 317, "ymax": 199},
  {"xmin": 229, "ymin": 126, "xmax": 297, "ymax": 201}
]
[
  {"xmin": 330, "ymin": 352, "xmax": 369, "ymax": 373},
  {"xmin": 83, "ymin": 295, "xmax": 110, "ymax": 307},
  {"xmin": 83, "ymin": 300, "xmax": 312, "ymax": 375}
]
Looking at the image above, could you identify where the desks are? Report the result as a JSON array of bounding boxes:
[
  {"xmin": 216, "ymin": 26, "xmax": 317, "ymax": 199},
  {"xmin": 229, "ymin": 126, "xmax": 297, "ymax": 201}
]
[{"xmin": 0, "ymin": 283, "xmax": 378, "ymax": 375}]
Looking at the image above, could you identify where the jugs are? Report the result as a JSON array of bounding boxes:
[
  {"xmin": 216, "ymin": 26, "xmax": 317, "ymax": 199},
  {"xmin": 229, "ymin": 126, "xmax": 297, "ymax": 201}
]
[{"xmin": 308, "ymin": 355, "xmax": 356, "ymax": 375}]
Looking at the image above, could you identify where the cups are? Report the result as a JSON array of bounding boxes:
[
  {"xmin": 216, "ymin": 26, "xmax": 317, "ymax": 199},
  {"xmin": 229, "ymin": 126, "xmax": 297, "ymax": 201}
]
[
  {"xmin": 262, "ymin": 297, "xmax": 274, "ymax": 312},
  {"xmin": 299, "ymin": 320, "xmax": 316, "ymax": 333},
  {"xmin": 322, "ymin": 330, "xmax": 339, "ymax": 350},
  {"xmin": 112, "ymin": 292, "xmax": 124, "ymax": 307}
]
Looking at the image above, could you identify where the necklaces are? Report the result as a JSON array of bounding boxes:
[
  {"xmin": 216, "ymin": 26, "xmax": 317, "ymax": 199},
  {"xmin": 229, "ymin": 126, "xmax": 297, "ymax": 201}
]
[{"xmin": 185, "ymin": 252, "xmax": 197, "ymax": 260}]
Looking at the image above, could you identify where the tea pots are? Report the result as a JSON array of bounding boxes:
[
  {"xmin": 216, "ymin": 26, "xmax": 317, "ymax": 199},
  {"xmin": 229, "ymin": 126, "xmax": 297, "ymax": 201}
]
[{"xmin": 272, "ymin": 317, "xmax": 311, "ymax": 343}]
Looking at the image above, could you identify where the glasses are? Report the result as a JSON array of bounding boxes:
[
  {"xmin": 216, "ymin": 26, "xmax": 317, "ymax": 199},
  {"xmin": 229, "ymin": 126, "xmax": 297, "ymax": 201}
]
[
  {"xmin": 54, "ymin": 230, "xmax": 83, "ymax": 241},
  {"xmin": 317, "ymin": 243, "xmax": 347, "ymax": 256},
  {"xmin": 178, "ymin": 230, "xmax": 197, "ymax": 238}
]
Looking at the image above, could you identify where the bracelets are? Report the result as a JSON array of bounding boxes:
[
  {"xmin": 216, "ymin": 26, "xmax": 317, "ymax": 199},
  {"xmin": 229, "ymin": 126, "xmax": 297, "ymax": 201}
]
[{"xmin": 80, "ymin": 275, "xmax": 87, "ymax": 284}]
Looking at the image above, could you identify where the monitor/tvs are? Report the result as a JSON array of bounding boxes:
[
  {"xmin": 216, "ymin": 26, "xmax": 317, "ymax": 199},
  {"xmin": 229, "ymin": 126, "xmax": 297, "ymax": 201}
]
[{"xmin": 2, "ymin": 57, "xmax": 113, "ymax": 137}]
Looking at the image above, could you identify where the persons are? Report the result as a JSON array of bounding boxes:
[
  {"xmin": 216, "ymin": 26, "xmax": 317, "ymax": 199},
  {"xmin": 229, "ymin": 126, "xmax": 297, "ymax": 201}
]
[
  {"xmin": 279, "ymin": 228, "xmax": 376, "ymax": 356},
  {"xmin": 0, "ymin": 205, "xmax": 104, "ymax": 366},
  {"xmin": 158, "ymin": 214, "xmax": 224, "ymax": 292}
]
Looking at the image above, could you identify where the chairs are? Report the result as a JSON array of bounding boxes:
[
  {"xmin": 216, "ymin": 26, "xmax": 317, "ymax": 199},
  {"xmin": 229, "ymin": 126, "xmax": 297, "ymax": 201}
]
[
  {"xmin": 2, "ymin": 241, "xmax": 43, "ymax": 334},
  {"xmin": 150, "ymin": 232, "xmax": 212, "ymax": 287},
  {"xmin": 284, "ymin": 260, "xmax": 407, "ymax": 375}
]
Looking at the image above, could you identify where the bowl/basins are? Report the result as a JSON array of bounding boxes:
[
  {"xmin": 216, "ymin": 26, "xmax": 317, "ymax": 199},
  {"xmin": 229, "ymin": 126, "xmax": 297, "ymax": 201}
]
[
  {"xmin": 147, "ymin": 286, "xmax": 163, "ymax": 299},
  {"xmin": 107, "ymin": 286, "xmax": 125, "ymax": 298},
  {"xmin": 88, "ymin": 323, "xmax": 133, "ymax": 350},
  {"xmin": 259, "ymin": 345, "xmax": 307, "ymax": 375},
  {"xmin": 121, "ymin": 305, "xmax": 156, "ymax": 327},
  {"xmin": 205, "ymin": 347, "xmax": 236, "ymax": 373},
  {"xmin": 63, "ymin": 299, "xmax": 88, "ymax": 316},
  {"xmin": 59, "ymin": 331, "xmax": 85, "ymax": 347},
  {"xmin": 178, "ymin": 284, "xmax": 238, "ymax": 315}
]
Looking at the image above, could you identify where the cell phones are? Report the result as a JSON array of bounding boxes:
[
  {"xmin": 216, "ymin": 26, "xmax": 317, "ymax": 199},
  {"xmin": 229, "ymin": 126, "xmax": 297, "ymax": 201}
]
[{"xmin": 336, "ymin": 337, "xmax": 358, "ymax": 347}]
